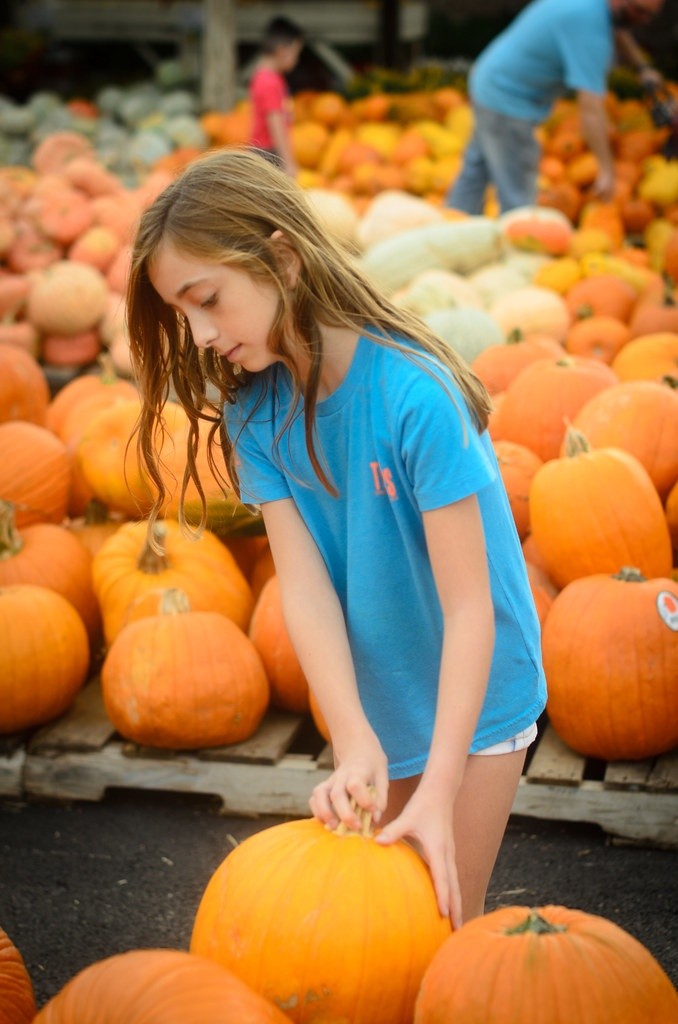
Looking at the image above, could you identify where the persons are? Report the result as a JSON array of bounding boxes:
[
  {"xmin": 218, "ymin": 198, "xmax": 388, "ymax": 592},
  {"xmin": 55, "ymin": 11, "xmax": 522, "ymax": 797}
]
[
  {"xmin": 249, "ymin": 15, "xmax": 305, "ymax": 173},
  {"xmin": 445, "ymin": 0, "xmax": 661, "ymax": 217},
  {"xmin": 123, "ymin": 149, "xmax": 547, "ymax": 932}
]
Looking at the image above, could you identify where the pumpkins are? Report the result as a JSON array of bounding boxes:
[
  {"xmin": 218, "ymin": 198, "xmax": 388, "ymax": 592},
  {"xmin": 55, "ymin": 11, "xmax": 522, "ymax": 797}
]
[
  {"xmin": 0, "ymin": 819, "xmax": 678, "ymax": 1024},
  {"xmin": 0, "ymin": 83, "xmax": 678, "ymax": 758}
]
